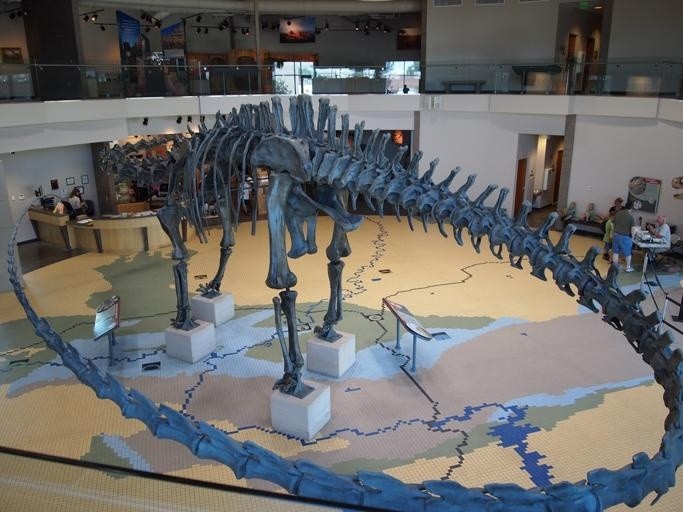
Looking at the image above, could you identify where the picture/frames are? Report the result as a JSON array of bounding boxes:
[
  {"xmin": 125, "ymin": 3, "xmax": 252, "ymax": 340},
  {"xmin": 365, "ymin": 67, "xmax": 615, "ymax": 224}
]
[{"xmin": 66, "ymin": 175, "xmax": 89, "ymax": 194}]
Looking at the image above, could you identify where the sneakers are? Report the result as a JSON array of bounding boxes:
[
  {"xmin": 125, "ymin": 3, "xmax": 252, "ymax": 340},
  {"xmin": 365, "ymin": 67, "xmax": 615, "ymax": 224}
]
[{"xmin": 625, "ymin": 267, "xmax": 634, "ymax": 272}]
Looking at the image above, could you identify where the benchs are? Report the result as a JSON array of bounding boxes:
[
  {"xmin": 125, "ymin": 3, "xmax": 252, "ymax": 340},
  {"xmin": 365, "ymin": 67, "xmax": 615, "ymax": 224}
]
[
  {"xmin": 561, "ymin": 217, "xmax": 605, "ymax": 240},
  {"xmin": 442, "ymin": 80, "xmax": 486, "ymax": 93}
]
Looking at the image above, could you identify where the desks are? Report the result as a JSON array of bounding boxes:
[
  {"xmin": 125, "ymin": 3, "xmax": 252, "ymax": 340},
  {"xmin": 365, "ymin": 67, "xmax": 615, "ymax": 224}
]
[{"xmin": 633, "ymin": 235, "xmax": 670, "ymax": 275}]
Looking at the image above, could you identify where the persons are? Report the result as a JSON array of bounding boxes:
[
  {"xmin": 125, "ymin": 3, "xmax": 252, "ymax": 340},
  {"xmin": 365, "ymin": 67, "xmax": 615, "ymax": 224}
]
[
  {"xmin": 68, "ymin": 189, "xmax": 88, "ymax": 210},
  {"xmin": 602, "ymin": 197, "xmax": 671, "ymax": 272},
  {"xmin": 239, "ymin": 180, "xmax": 252, "ymax": 207}
]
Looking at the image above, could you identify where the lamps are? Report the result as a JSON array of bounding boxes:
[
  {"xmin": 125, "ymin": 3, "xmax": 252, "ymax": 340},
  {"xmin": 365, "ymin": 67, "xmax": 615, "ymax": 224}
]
[
  {"xmin": 196, "ymin": 13, "xmax": 254, "ymax": 35},
  {"xmin": 4, "ymin": 0, "xmax": 32, "ymax": 19},
  {"xmin": 79, "ymin": 7, "xmax": 107, "ymax": 32},
  {"xmin": 351, "ymin": 17, "xmax": 380, "ymax": 33},
  {"xmin": 260, "ymin": 19, "xmax": 298, "ymax": 34},
  {"xmin": 139, "ymin": 7, "xmax": 163, "ymax": 33},
  {"xmin": 313, "ymin": 18, "xmax": 331, "ymax": 34}
]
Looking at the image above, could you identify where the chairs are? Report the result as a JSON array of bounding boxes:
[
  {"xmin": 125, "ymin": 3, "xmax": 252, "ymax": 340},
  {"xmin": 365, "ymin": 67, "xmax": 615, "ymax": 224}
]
[{"xmin": 656, "ymin": 234, "xmax": 680, "ymax": 267}]
